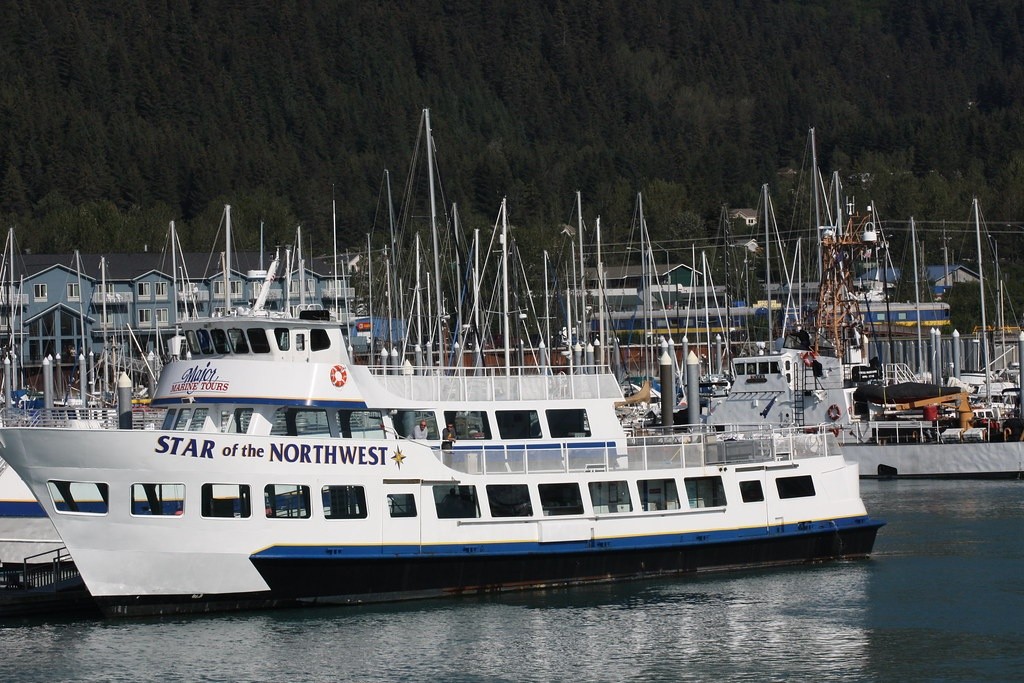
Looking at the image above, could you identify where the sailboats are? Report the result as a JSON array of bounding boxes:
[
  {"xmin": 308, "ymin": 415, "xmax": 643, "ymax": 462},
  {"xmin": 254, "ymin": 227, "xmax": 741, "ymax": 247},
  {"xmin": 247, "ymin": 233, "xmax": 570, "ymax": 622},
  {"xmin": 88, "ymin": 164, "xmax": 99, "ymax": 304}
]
[
  {"xmin": 685, "ymin": 128, "xmax": 1024, "ymax": 478},
  {"xmin": 3, "ymin": 108, "xmax": 885, "ymax": 619},
  {"xmin": 1, "ymin": 167, "xmax": 1021, "ymax": 568}
]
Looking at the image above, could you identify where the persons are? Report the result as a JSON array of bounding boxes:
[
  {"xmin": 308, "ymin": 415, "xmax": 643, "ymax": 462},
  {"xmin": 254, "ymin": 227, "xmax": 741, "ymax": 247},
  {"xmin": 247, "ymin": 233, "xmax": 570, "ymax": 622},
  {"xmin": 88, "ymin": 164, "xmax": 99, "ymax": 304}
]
[
  {"xmin": 69, "ymin": 345, "xmax": 77, "ymax": 362},
  {"xmin": 442, "ymin": 423, "xmax": 456, "ymax": 468},
  {"xmin": 406, "ymin": 419, "xmax": 428, "ymax": 439}
]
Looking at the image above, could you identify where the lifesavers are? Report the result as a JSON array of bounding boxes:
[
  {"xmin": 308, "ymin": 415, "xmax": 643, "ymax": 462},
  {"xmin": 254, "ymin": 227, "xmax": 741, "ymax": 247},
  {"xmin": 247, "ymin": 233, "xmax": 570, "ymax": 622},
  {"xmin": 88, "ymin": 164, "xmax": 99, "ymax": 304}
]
[
  {"xmin": 329, "ymin": 364, "xmax": 348, "ymax": 387},
  {"xmin": 827, "ymin": 404, "xmax": 842, "ymax": 421},
  {"xmin": 803, "ymin": 351, "xmax": 816, "ymax": 368}
]
